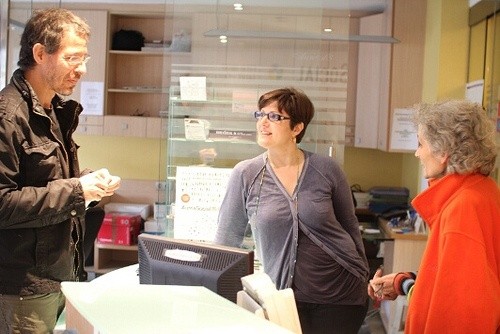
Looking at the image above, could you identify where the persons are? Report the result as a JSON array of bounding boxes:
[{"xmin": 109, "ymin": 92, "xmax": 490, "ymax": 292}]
[
  {"xmin": 0, "ymin": 7, "xmax": 122, "ymax": 334},
  {"xmin": 214, "ymin": 86, "xmax": 369, "ymax": 334},
  {"xmin": 368, "ymin": 100, "xmax": 500, "ymax": 334}
]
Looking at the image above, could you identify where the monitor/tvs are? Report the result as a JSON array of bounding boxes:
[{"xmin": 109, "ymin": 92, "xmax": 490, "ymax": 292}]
[{"xmin": 137, "ymin": 232, "xmax": 254, "ymax": 304}]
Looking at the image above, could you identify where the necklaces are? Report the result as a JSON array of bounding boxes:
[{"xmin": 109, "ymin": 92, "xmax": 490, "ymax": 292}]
[{"xmin": 253, "ymin": 155, "xmax": 300, "ymax": 245}]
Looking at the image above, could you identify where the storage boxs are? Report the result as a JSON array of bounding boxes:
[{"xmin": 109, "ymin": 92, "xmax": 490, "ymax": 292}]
[
  {"xmin": 99, "ymin": 214, "xmax": 142, "ymax": 246},
  {"xmin": 102, "ymin": 203, "xmax": 152, "ymax": 218}
]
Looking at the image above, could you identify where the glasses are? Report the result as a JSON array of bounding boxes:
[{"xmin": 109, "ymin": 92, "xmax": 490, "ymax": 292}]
[
  {"xmin": 254, "ymin": 111, "xmax": 291, "ymax": 121},
  {"xmin": 63, "ymin": 55, "xmax": 92, "ymax": 66}
]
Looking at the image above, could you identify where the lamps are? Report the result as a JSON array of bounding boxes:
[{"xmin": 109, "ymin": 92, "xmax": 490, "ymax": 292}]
[{"xmin": 204, "ymin": 0, "xmax": 401, "ymax": 44}]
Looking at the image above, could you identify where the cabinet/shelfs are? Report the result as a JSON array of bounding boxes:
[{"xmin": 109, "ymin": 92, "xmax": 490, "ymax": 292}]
[
  {"xmin": 352, "ymin": 0, "xmax": 425, "ymax": 153},
  {"xmin": 377, "ymin": 220, "xmax": 427, "ymax": 334},
  {"xmin": 85, "ymin": 177, "xmax": 176, "ymax": 274},
  {"xmin": 105, "ymin": 5, "xmax": 191, "ymax": 138},
  {"xmin": 191, "ymin": 7, "xmax": 377, "ymax": 143},
  {"xmin": 62, "ymin": 3, "xmax": 116, "ymax": 133}
]
